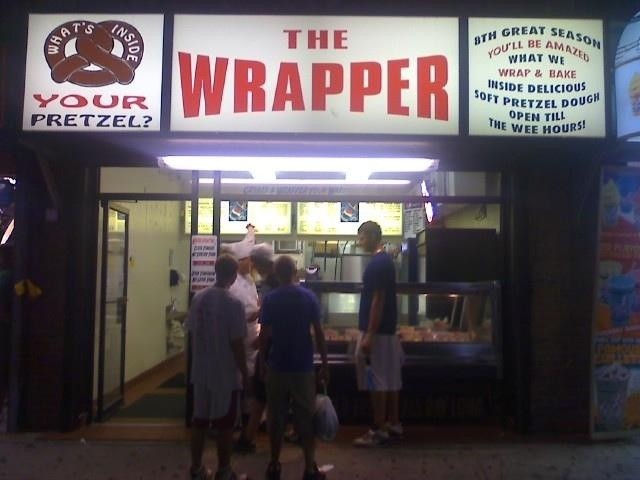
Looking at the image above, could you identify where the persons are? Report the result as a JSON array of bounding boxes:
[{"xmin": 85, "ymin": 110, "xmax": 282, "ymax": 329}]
[
  {"xmin": 352, "ymin": 220, "xmax": 404, "ymax": 448},
  {"xmin": 183, "ymin": 242, "xmax": 332, "ymax": 480}
]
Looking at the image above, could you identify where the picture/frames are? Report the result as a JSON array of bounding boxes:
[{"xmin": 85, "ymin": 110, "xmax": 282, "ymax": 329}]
[
  {"xmin": 340, "ymin": 201, "xmax": 359, "ymax": 222},
  {"xmin": 229, "ymin": 200, "xmax": 247, "ymax": 221}
]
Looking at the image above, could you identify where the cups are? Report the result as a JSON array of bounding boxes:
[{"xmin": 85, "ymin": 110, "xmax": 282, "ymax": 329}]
[
  {"xmin": 230, "ymin": 202, "xmax": 246, "ymax": 220},
  {"xmin": 342, "ymin": 203, "xmax": 358, "ymax": 222}
]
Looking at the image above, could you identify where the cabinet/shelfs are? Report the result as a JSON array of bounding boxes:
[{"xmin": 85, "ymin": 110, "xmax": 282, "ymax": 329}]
[
  {"xmin": 291, "ymin": 279, "xmax": 510, "ymax": 424},
  {"xmin": 401, "ymin": 225, "xmax": 496, "ymax": 322}
]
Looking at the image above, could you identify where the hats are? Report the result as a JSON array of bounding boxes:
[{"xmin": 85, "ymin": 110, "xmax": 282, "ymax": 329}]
[{"xmin": 231, "ymin": 242, "xmax": 251, "ymax": 260}]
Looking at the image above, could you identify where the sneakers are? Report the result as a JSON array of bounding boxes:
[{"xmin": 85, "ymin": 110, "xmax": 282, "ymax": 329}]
[
  {"xmin": 213, "ymin": 469, "xmax": 247, "ymax": 480},
  {"xmin": 351, "ymin": 422, "xmax": 403, "ymax": 448},
  {"xmin": 302, "ymin": 461, "xmax": 326, "ymax": 480},
  {"xmin": 283, "ymin": 428, "xmax": 301, "ymax": 444},
  {"xmin": 189, "ymin": 463, "xmax": 213, "ymax": 479},
  {"xmin": 234, "ymin": 436, "xmax": 256, "ymax": 452},
  {"xmin": 265, "ymin": 462, "xmax": 281, "ymax": 479}
]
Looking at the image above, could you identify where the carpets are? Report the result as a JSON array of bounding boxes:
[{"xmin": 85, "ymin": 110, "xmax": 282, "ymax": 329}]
[{"xmin": 110, "ymin": 368, "xmax": 186, "ymax": 424}]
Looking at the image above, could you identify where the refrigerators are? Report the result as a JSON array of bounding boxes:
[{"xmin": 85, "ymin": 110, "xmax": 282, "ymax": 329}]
[
  {"xmin": 399, "ymin": 237, "xmax": 415, "ymax": 325},
  {"xmin": 414, "ymin": 227, "xmax": 497, "ymax": 317}
]
